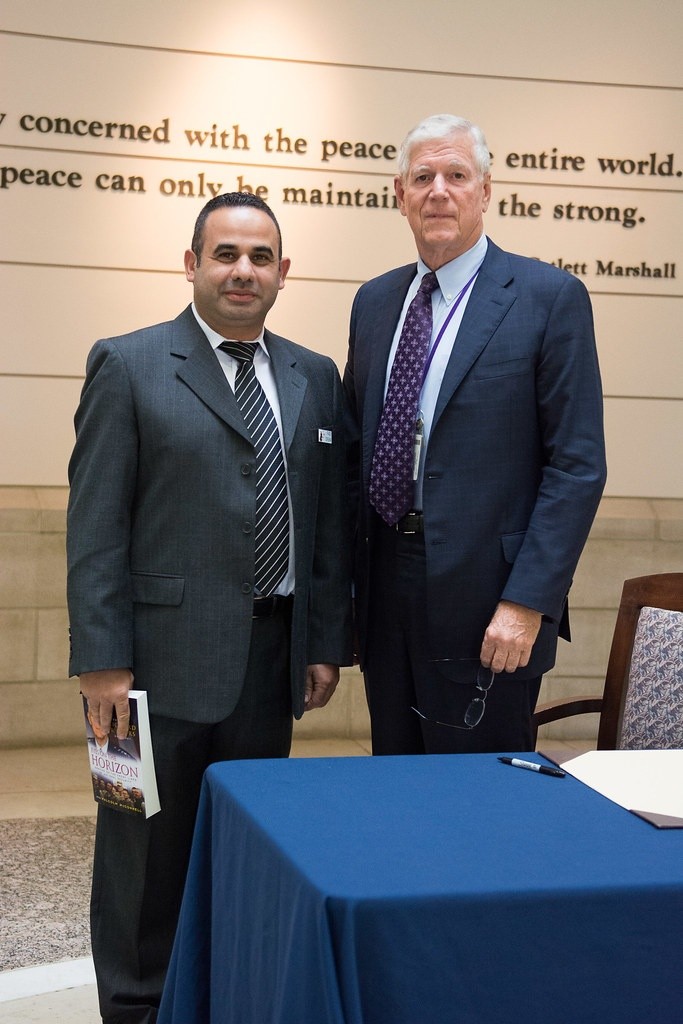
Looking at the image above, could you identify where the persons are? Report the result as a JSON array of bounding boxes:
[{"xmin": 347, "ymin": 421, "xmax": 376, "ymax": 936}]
[
  {"xmin": 66, "ymin": 191, "xmax": 353, "ymax": 1024},
  {"xmin": 86, "ymin": 706, "xmax": 137, "ymax": 762},
  {"xmin": 92, "ymin": 774, "xmax": 145, "ymax": 814},
  {"xmin": 342, "ymin": 115, "xmax": 607, "ymax": 756}
]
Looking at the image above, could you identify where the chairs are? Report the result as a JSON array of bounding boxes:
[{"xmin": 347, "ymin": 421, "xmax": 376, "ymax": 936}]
[{"xmin": 534, "ymin": 572, "xmax": 683, "ymax": 750}]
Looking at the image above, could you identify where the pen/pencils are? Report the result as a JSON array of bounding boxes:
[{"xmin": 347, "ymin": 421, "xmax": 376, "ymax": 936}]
[{"xmin": 497, "ymin": 756, "xmax": 566, "ymax": 778}]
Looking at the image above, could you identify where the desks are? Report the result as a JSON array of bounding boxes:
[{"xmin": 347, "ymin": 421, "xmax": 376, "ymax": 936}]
[{"xmin": 154, "ymin": 752, "xmax": 683, "ymax": 1023}]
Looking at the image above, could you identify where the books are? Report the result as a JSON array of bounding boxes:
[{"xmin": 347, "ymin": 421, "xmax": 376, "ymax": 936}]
[{"xmin": 81, "ymin": 689, "xmax": 162, "ymax": 819}]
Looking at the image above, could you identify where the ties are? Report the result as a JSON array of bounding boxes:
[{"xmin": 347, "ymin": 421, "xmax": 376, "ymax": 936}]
[
  {"xmin": 219, "ymin": 338, "xmax": 292, "ymax": 599},
  {"xmin": 368, "ymin": 271, "xmax": 439, "ymax": 526}
]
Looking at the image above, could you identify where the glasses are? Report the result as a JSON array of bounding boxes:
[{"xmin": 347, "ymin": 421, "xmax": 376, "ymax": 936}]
[{"xmin": 412, "ymin": 658, "xmax": 495, "ymax": 730}]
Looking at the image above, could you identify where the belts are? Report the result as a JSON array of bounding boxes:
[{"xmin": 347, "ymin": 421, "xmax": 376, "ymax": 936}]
[
  {"xmin": 378, "ymin": 509, "xmax": 429, "ymax": 535},
  {"xmin": 252, "ymin": 595, "xmax": 294, "ymax": 619}
]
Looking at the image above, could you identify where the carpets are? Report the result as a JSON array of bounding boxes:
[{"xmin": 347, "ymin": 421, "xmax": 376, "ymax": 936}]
[{"xmin": 0, "ymin": 790, "xmax": 97, "ymax": 1001}]
